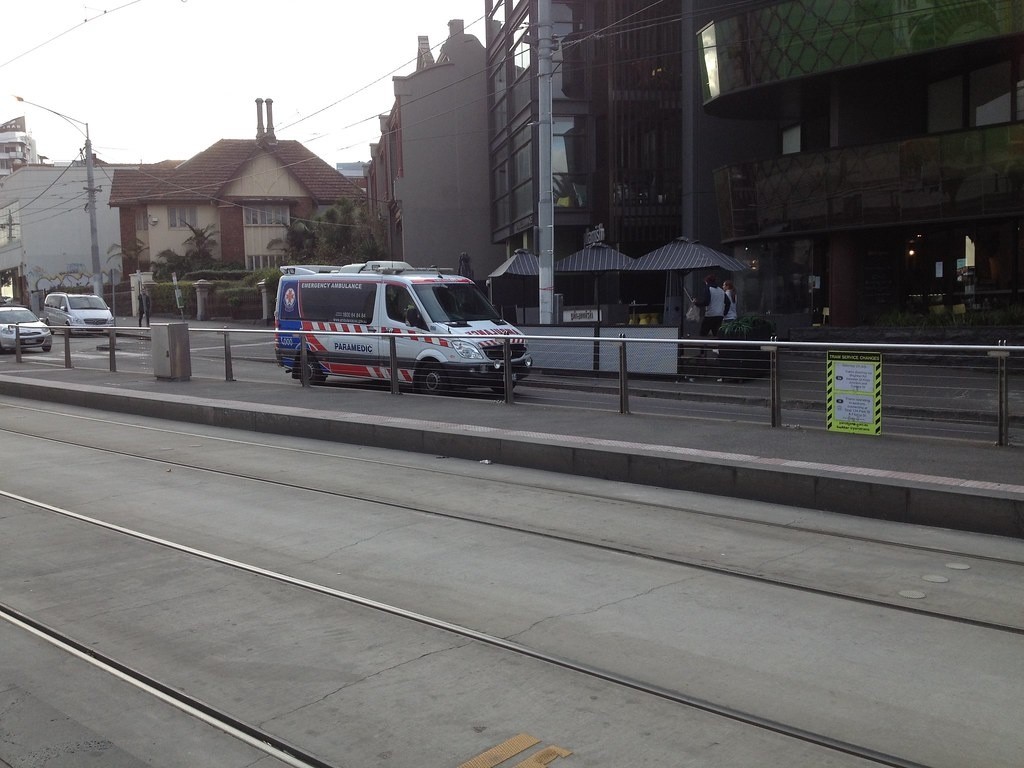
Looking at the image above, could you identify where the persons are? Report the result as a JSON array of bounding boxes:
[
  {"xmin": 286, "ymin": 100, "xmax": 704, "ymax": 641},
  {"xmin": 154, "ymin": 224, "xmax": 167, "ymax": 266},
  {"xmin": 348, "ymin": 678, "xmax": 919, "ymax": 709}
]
[
  {"xmin": 712, "ymin": 280, "xmax": 737, "ymax": 354},
  {"xmin": 138, "ymin": 290, "xmax": 150, "ymax": 326},
  {"xmin": 694, "ymin": 275, "xmax": 731, "ymax": 357}
]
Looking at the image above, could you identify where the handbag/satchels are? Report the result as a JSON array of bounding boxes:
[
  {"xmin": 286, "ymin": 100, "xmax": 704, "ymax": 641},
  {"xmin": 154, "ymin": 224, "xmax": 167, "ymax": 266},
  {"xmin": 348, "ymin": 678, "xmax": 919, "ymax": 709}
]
[{"xmin": 686, "ymin": 298, "xmax": 700, "ymax": 323}]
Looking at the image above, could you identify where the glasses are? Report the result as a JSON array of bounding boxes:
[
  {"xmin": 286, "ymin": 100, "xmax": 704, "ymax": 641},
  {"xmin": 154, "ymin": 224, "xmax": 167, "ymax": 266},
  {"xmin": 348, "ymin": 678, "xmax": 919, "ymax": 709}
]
[{"xmin": 142, "ymin": 291, "xmax": 146, "ymax": 292}]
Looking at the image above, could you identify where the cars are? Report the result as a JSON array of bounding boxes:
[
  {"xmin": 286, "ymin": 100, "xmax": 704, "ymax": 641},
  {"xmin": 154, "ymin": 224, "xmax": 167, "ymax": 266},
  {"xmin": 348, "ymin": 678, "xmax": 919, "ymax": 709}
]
[
  {"xmin": 0, "ymin": 305, "xmax": 51, "ymax": 353},
  {"xmin": 43, "ymin": 291, "xmax": 116, "ymax": 335}
]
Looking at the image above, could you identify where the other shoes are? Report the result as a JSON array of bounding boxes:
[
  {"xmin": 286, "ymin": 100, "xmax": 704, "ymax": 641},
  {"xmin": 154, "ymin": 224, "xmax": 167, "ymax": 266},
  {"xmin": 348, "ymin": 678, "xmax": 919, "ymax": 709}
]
[
  {"xmin": 693, "ymin": 353, "xmax": 704, "ymax": 358},
  {"xmin": 713, "ymin": 349, "xmax": 719, "ymax": 354}
]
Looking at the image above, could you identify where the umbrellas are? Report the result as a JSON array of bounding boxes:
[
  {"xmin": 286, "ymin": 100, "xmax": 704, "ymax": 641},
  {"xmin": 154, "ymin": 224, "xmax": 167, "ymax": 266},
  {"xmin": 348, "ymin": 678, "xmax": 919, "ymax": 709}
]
[
  {"xmin": 488, "ymin": 250, "xmax": 539, "ymax": 324},
  {"xmin": 620, "ymin": 237, "xmax": 748, "ymax": 357},
  {"xmin": 458, "ymin": 252, "xmax": 471, "ymax": 279},
  {"xmin": 553, "ymin": 243, "xmax": 637, "ymax": 324}
]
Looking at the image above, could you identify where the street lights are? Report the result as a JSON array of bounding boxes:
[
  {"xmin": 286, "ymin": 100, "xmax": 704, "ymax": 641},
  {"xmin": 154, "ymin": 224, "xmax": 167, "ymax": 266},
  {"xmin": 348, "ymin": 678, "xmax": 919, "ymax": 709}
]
[{"xmin": 15, "ymin": 96, "xmax": 103, "ymax": 299}]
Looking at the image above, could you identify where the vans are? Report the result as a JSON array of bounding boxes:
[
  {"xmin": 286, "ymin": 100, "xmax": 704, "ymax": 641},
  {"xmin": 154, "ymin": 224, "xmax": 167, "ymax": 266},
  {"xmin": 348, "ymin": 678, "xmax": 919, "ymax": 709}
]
[{"xmin": 272, "ymin": 258, "xmax": 534, "ymax": 391}]
